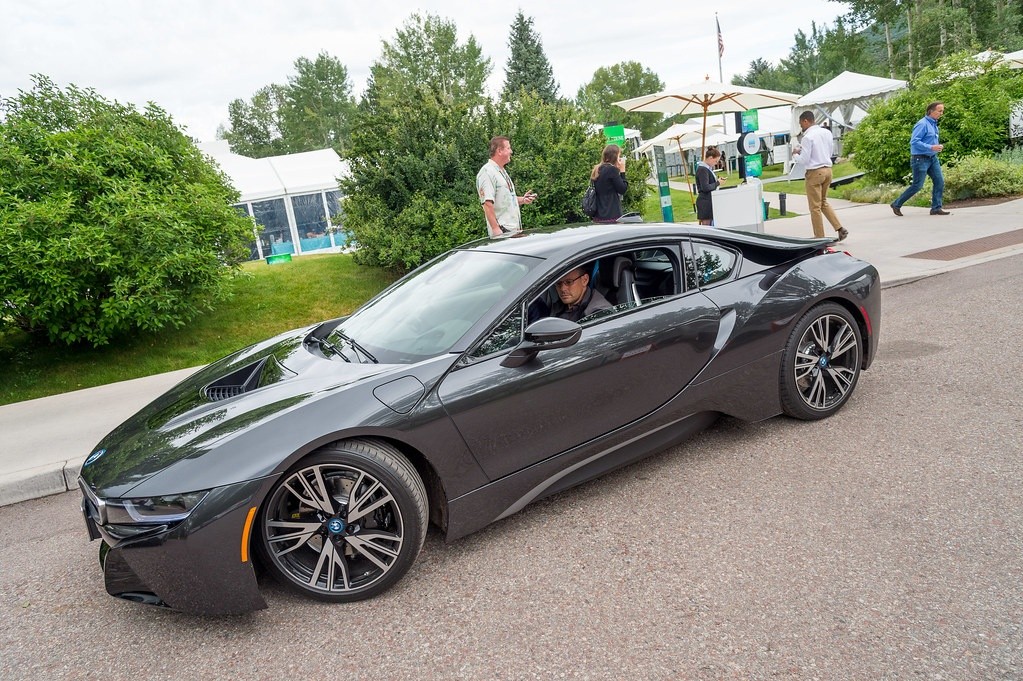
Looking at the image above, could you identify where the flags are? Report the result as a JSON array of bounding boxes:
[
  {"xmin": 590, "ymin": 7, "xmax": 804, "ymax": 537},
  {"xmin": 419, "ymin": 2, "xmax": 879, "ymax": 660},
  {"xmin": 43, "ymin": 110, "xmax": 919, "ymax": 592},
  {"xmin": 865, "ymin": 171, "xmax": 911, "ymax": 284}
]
[{"xmin": 717, "ymin": 21, "xmax": 724, "ymax": 57}]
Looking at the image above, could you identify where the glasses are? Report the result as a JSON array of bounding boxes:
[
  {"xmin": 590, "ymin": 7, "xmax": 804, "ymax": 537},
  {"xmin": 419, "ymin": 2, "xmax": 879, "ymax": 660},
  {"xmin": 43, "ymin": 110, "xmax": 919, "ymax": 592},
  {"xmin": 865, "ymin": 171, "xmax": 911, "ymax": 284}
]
[{"xmin": 555, "ymin": 273, "xmax": 586, "ymax": 287}]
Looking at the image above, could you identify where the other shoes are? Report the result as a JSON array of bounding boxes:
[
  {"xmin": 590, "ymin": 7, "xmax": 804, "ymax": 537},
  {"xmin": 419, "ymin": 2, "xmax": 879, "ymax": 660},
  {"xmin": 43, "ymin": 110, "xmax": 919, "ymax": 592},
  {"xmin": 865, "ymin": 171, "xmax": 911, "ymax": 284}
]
[
  {"xmin": 930, "ymin": 209, "xmax": 950, "ymax": 215},
  {"xmin": 838, "ymin": 227, "xmax": 849, "ymax": 241},
  {"xmin": 891, "ymin": 204, "xmax": 903, "ymax": 216}
]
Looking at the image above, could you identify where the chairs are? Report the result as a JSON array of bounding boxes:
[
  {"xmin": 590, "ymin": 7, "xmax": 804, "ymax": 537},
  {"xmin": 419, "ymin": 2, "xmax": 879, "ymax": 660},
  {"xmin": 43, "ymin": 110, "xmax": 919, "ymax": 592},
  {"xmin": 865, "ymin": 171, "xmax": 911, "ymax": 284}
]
[{"xmin": 596, "ymin": 252, "xmax": 638, "ymax": 305}]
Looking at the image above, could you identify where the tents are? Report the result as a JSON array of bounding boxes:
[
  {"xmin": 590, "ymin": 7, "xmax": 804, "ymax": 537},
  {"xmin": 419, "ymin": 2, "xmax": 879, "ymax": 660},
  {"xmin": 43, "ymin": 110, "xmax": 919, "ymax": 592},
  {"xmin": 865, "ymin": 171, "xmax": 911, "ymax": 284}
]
[{"xmin": 791, "ymin": 70, "xmax": 909, "ymax": 159}]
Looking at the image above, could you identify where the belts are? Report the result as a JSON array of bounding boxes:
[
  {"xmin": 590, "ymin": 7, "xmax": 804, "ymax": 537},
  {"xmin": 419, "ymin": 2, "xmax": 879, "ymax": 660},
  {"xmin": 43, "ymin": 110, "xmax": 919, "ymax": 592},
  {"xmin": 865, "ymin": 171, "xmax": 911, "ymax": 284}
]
[{"xmin": 912, "ymin": 154, "xmax": 935, "ymax": 159}]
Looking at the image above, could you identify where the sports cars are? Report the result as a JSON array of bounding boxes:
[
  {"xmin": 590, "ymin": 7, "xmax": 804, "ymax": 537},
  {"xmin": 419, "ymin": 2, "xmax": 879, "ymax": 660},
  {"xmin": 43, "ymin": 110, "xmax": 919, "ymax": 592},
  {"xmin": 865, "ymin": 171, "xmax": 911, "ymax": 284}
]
[{"xmin": 75, "ymin": 218, "xmax": 883, "ymax": 620}]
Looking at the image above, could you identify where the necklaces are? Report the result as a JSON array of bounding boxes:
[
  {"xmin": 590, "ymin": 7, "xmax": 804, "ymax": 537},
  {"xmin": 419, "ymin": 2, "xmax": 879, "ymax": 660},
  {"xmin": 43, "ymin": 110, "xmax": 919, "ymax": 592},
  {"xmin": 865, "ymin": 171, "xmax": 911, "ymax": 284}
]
[{"xmin": 500, "ymin": 171, "xmax": 514, "ymax": 191}]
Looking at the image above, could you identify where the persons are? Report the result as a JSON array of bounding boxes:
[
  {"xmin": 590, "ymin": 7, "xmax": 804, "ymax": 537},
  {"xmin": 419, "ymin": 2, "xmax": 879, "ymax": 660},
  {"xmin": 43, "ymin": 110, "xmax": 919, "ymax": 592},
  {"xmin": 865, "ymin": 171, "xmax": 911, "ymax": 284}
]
[
  {"xmin": 695, "ymin": 146, "xmax": 726, "ymax": 227},
  {"xmin": 792, "ymin": 110, "xmax": 849, "ymax": 242},
  {"xmin": 305, "ymin": 224, "xmax": 326, "ymax": 237},
  {"xmin": 476, "ymin": 137, "xmax": 537, "ymax": 237},
  {"xmin": 553, "ymin": 266, "xmax": 615, "ymax": 324},
  {"xmin": 821, "ymin": 122, "xmax": 831, "ymax": 130},
  {"xmin": 890, "ymin": 102, "xmax": 949, "ymax": 216},
  {"xmin": 721, "ymin": 151, "xmax": 726, "ymax": 161},
  {"xmin": 591, "ymin": 144, "xmax": 628, "ymax": 223},
  {"xmin": 499, "ymin": 264, "xmax": 550, "ymax": 327}
]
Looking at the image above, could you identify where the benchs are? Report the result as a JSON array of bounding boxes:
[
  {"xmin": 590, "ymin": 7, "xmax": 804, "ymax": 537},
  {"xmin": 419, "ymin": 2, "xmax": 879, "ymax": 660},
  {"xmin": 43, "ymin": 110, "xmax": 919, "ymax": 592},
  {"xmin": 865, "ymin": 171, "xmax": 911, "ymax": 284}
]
[{"xmin": 829, "ymin": 171, "xmax": 873, "ymax": 189}]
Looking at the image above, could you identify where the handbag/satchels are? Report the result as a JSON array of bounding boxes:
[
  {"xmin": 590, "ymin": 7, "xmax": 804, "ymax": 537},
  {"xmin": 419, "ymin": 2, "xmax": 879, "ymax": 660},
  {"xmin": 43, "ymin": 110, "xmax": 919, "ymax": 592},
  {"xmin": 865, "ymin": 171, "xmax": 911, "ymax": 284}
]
[{"xmin": 581, "ymin": 180, "xmax": 596, "ymax": 216}]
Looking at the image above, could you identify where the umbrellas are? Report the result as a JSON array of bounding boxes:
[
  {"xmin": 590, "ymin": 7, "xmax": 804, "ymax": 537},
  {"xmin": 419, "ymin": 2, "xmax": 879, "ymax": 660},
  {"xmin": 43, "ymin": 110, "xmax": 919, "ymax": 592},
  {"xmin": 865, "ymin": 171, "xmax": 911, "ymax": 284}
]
[
  {"xmin": 926, "ymin": 46, "xmax": 1023, "ymax": 86},
  {"xmin": 612, "ymin": 76, "xmax": 803, "ymax": 162},
  {"xmin": 630, "ymin": 122, "xmax": 737, "ymax": 214}
]
